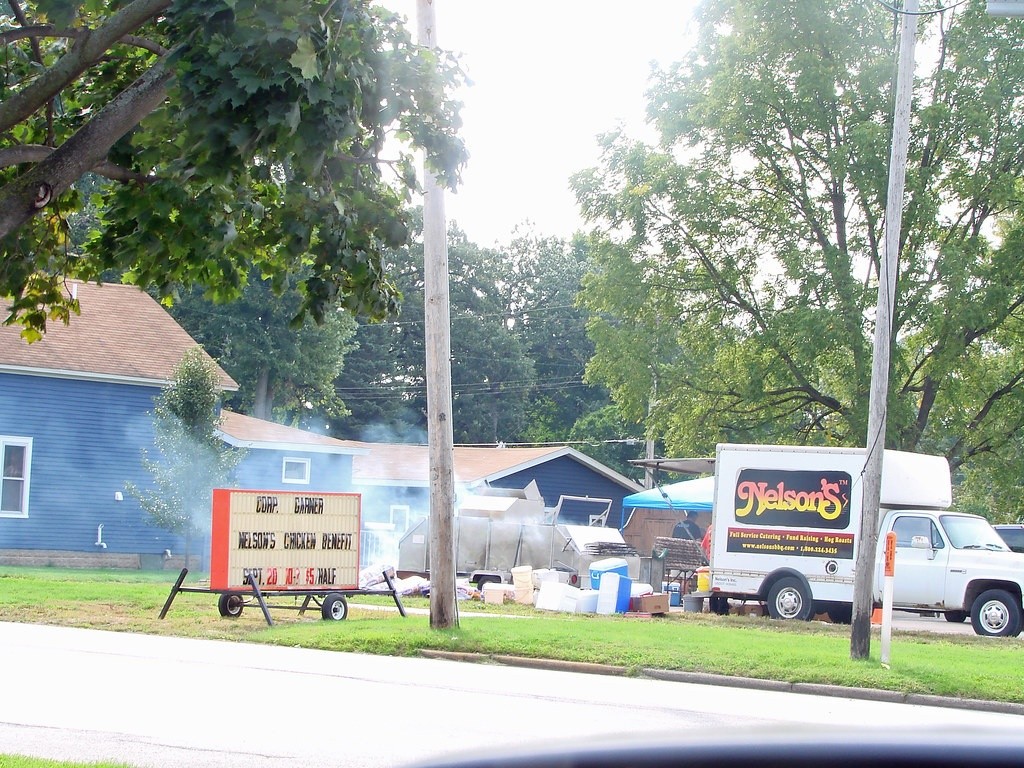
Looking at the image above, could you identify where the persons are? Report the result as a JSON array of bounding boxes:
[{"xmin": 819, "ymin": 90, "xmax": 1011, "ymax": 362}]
[
  {"xmin": 701, "ymin": 521, "xmax": 712, "ymax": 565},
  {"xmin": 672, "ymin": 511, "xmax": 701, "ymax": 597}
]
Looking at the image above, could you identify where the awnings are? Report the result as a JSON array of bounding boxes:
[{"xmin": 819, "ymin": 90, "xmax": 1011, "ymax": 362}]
[{"xmin": 628, "ymin": 458, "xmax": 716, "ymax": 563}]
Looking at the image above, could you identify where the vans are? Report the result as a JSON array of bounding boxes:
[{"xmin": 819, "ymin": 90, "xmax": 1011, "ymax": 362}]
[{"xmin": 991, "ymin": 524, "xmax": 1024, "ymax": 554}]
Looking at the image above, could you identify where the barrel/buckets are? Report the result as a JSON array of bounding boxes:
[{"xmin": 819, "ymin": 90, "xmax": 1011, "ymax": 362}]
[
  {"xmin": 682, "ymin": 596, "xmax": 704, "ymax": 612},
  {"xmin": 511, "ymin": 565, "xmax": 533, "ymax": 604},
  {"xmin": 483, "ymin": 583, "xmax": 505, "ymax": 605}
]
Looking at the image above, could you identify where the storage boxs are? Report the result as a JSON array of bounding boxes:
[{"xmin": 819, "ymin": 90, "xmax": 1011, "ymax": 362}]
[
  {"xmin": 740, "ymin": 601, "xmax": 763, "ymax": 616},
  {"xmin": 483, "ymin": 583, "xmax": 504, "ymax": 604},
  {"xmin": 533, "ymin": 558, "xmax": 680, "ymax": 614}
]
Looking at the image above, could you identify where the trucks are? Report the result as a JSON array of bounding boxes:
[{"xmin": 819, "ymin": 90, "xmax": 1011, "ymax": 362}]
[{"xmin": 626, "ymin": 441, "xmax": 1024, "ymax": 638}]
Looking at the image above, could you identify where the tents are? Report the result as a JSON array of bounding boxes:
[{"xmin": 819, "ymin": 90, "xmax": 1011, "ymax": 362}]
[{"xmin": 621, "ymin": 476, "xmax": 714, "ymax": 537}]
